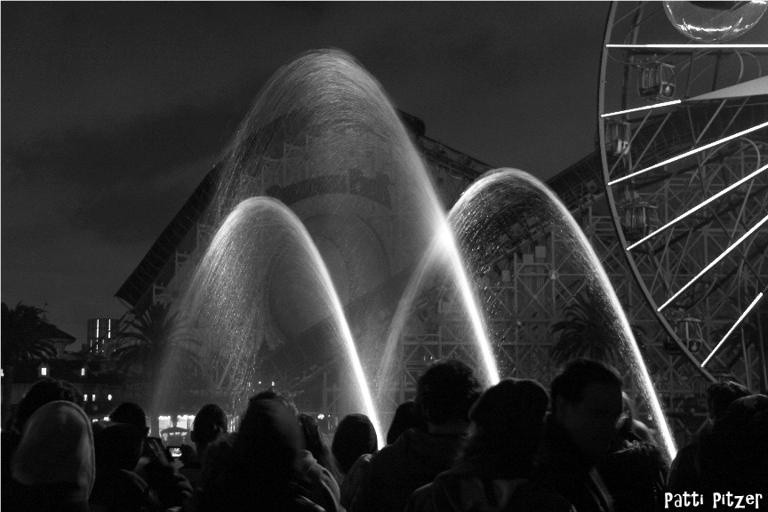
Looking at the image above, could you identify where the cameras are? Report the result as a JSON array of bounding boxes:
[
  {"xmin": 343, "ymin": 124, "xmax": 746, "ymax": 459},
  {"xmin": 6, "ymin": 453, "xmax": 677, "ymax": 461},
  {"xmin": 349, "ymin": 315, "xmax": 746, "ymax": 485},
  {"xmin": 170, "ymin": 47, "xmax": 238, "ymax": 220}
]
[{"xmin": 167, "ymin": 446, "xmax": 182, "ymax": 459}]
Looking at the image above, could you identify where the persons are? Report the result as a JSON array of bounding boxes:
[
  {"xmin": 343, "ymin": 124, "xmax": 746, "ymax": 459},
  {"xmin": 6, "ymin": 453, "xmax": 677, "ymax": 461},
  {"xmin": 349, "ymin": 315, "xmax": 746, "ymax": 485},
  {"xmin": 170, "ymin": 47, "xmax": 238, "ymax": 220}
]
[{"xmin": 2, "ymin": 354, "xmax": 766, "ymax": 512}]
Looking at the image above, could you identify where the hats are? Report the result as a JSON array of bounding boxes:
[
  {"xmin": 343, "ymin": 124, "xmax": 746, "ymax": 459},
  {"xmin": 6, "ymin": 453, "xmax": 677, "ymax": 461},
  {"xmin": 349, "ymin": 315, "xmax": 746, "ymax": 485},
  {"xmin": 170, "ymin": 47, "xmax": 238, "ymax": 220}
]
[{"xmin": 469, "ymin": 377, "xmax": 548, "ymax": 425}]
